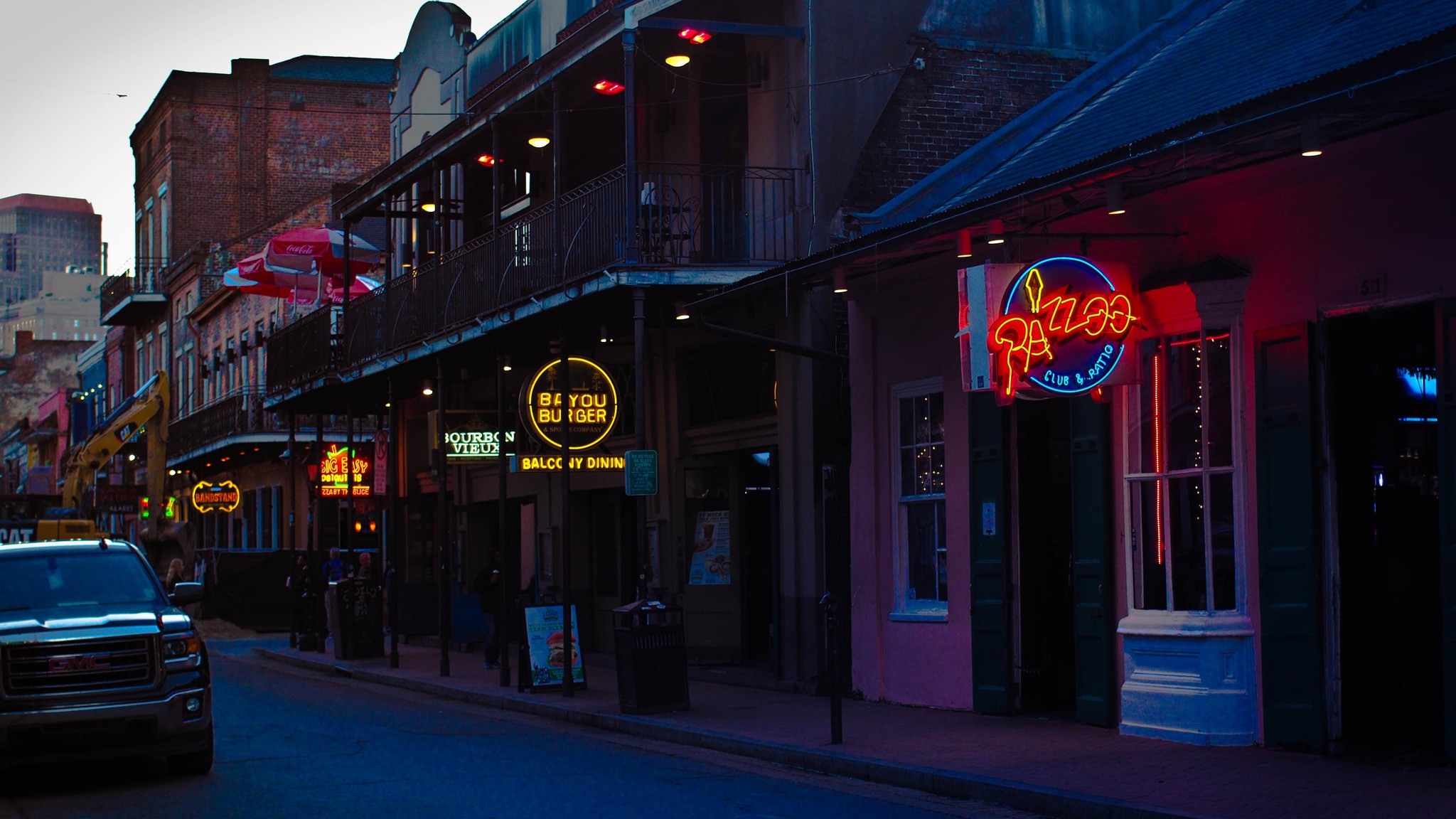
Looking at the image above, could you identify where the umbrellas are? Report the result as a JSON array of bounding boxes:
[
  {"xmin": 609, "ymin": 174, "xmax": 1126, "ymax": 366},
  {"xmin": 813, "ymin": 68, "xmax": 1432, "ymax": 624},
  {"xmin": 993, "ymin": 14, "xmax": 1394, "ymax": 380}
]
[{"xmin": 222, "ymin": 227, "xmax": 384, "ymax": 333}]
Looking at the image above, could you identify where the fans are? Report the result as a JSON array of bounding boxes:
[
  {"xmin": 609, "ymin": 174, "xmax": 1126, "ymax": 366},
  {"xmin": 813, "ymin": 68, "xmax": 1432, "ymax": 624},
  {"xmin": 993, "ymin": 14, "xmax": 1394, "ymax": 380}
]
[{"xmin": 392, "ymin": 180, "xmax": 465, "ymax": 211}]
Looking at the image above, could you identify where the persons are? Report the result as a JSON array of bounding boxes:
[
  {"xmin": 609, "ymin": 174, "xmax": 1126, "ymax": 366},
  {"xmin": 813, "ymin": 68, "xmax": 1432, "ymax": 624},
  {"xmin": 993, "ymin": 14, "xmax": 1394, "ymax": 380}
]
[
  {"xmin": 165, "ymin": 559, "xmax": 183, "ymax": 592},
  {"xmin": 476, "ymin": 547, "xmax": 503, "ymax": 669},
  {"xmin": 286, "ymin": 548, "xmax": 376, "ymax": 589}
]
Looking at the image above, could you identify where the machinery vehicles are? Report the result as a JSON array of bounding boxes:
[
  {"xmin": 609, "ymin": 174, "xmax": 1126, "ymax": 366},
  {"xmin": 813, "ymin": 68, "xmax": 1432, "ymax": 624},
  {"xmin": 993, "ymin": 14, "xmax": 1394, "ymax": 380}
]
[{"xmin": 0, "ymin": 369, "xmax": 196, "ymax": 609}]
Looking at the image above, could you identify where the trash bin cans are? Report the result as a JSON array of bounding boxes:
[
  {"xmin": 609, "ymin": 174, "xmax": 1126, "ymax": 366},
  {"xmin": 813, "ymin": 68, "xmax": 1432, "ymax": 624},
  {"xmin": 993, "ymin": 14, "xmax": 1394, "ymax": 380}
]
[
  {"xmin": 326, "ymin": 574, "xmax": 385, "ymax": 657},
  {"xmin": 611, "ymin": 601, "xmax": 693, "ymax": 715}
]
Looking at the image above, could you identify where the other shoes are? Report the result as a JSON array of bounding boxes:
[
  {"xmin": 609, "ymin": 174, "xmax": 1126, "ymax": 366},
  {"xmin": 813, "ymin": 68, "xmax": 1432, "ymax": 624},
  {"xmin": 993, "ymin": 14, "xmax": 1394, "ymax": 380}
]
[{"xmin": 486, "ymin": 654, "xmax": 500, "ymax": 668}]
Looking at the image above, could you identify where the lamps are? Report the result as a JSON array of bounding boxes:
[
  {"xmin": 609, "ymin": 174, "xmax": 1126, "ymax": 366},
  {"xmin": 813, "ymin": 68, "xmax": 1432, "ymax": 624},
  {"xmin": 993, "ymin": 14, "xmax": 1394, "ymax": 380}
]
[
  {"xmin": 601, "ymin": 326, "xmax": 607, "ymax": 343},
  {"xmin": 529, "ymin": 170, "xmax": 541, "ymax": 198},
  {"xmin": 674, "ymin": 303, "xmax": 689, "ymax": 321},
  {"xmin": 1298, "ymin": 117, "xmax": 1322, "ymax": 157},
  {"xmin": 747, "ymin": 49, "xmax": 770, "ymax": 90},
  {"xmin": 957, "ymin": 227, "xmax": 973, "ymax": 258},
  {"xmin": 833, "ymin": 265, "xmax": 849, "ymax": 295},
  {"xmin": 503, "ymin": 354, "xmax": 513, "ymax": 372},
  {"xmin": 1103, "ymin": 180, "xmax": 1126, "ymax": 215},
  {"xmin": 421, "ymin": 380, "xmax": 433, "ymax": 396},
  {"xmin": 657, "ymin": 98, "xmax": 676, "ymax": 131},
  {"xmin": 986, "ymin": 219, "xmax": 1005, "ymax": 245}
]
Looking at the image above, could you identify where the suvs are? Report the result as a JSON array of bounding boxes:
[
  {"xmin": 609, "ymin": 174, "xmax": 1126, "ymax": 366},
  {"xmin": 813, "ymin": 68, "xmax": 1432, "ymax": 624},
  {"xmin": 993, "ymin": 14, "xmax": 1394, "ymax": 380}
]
[{"xmin": 1, "ymin": 536, "xmax": 214, "ymax": 800}]
